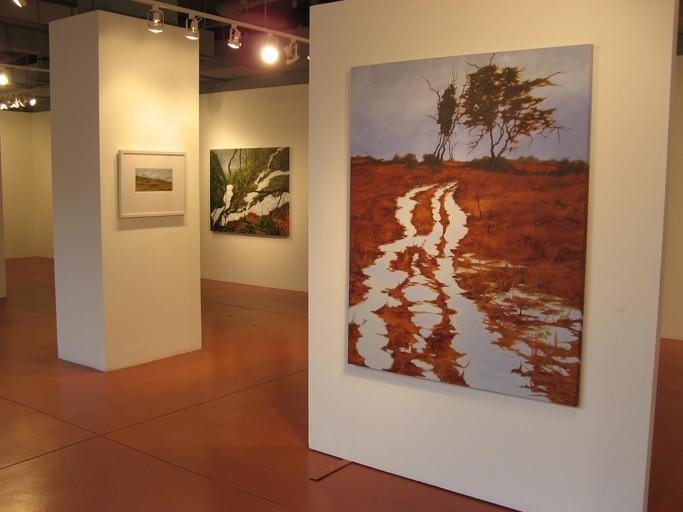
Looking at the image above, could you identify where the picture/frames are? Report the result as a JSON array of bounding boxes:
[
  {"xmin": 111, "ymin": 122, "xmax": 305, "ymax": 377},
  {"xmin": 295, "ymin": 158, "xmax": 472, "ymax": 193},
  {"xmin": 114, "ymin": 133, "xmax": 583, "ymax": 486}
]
[{"xmin": 116, "ymin": 150, "xmax": 187, "ymax": 219}]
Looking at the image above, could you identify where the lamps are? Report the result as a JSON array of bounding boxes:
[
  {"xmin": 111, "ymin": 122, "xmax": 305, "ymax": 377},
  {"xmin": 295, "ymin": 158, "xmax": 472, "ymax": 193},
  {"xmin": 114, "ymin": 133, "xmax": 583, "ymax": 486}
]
[{"xmin": 145, "ymin": 4, "xmax": 246, "ymax": 50}]
